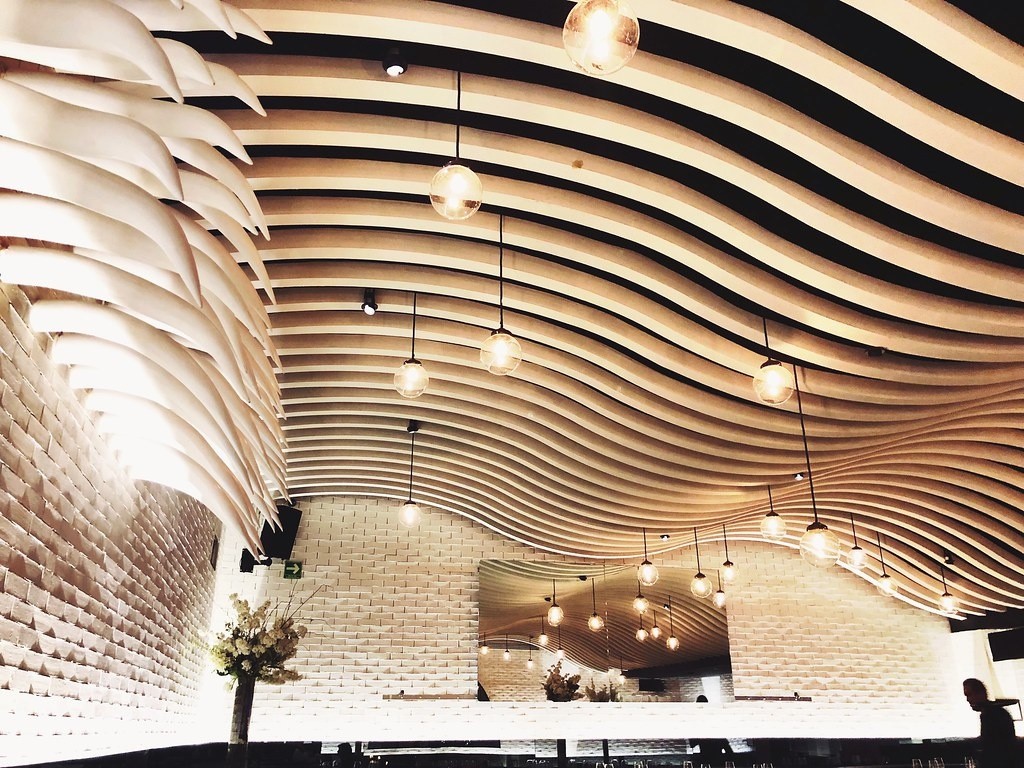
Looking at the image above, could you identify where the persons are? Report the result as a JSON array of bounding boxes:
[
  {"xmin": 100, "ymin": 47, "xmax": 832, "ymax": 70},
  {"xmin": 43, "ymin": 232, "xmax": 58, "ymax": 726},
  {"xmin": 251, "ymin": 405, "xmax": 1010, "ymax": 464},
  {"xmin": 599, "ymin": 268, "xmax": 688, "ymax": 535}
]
[
  {"xmin": 687, "ymin": 695, "xmax": 732, "ymax": 753},
  {"xmin": 964, "ymin": 678, "xmax": 1014, "ymax": 768}
]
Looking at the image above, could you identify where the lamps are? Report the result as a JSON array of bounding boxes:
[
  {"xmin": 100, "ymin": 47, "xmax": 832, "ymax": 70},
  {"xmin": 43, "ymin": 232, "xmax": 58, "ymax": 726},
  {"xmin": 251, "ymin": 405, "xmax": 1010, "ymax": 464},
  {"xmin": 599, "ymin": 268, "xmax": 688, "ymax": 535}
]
[
  {"xmin": 562, "ymin": 0, "xmax": 640, "ymax": 77},
  {"xmin": 394, "ymin": 292, "xmax": 430, "ymax": 397},
  {"xmin": 428, "ymin": 63, "xmax": 483, "ymax": 221},
  {"xmin": 875, "ymin": 531, "xmax": 898, "ymax": 597},
  {"xmin": 848, "ymin": 513, "xmax": 868, "ymax": 570},
  {"xmin": 480, "ymin": 523, "xmax": 735, "ymax": 670},
  {"xmin": 790, "ymin": 363, "xmax": 841, "ymax": 569},
  {"xmin": 399, "ymin": 431, "xmax": 422, "ymax": 527},
  {"xmin": 480, "ymin": 211, "xmax": 524, "ymax": 375},
  {"xmin": 752, "ymin": 309, "xmax": 795, "ymax": 404},
  {"xmin": 760, "ymin": 485, "xmax": 787, "ymax": 542},
  {"xmin": 939, "ymin": 562, "xmax": 961, "ymax": 616}
]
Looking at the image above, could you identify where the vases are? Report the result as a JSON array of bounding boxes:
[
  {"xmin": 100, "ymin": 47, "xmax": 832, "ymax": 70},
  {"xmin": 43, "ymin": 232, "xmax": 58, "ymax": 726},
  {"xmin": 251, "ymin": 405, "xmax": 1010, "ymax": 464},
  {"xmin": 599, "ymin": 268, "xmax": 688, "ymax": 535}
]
[{"xmin": 225, "ymin": 678, "xmax": 257, "ymax": 748}]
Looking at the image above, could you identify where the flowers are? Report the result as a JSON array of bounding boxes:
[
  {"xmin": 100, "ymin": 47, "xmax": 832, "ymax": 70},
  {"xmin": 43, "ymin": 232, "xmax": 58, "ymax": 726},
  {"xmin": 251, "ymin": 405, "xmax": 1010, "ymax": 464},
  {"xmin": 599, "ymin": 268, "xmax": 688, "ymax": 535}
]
[{"xmin": 191, "ymin": 575, "xmax": 333, "ymax": 692}]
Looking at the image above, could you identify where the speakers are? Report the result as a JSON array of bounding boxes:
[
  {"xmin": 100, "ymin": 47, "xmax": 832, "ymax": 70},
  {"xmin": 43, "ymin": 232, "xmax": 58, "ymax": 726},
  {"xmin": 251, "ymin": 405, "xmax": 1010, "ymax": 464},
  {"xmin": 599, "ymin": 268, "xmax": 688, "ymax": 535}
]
[{"xmin": 257, "ymin": 505, "xmax": 301, "ymax": 559}]
